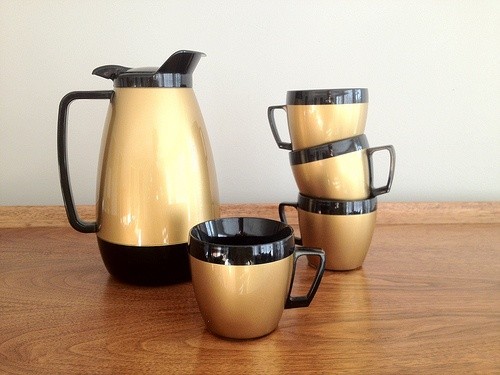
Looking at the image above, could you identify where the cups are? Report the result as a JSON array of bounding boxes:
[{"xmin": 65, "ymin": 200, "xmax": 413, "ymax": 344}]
[
  {"xmin": 189, "ymin": 217, "xmax": 326, "ymax": 339},
  {"xmin": 267, "ymin": 88, "xmax": 369, "ymax": 151},
  {"xmin": 278, "ymin": 192, "xmax": 377, "ymax": 271},
  {"xmin": 289, "ymin": 134, "xmax": 395, "ymax": 201}
]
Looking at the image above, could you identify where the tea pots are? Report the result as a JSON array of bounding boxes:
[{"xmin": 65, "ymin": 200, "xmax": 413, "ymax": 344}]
[{"xmin": 57, "ymin": 50, "xmax": 220, "ymax": 286}]
